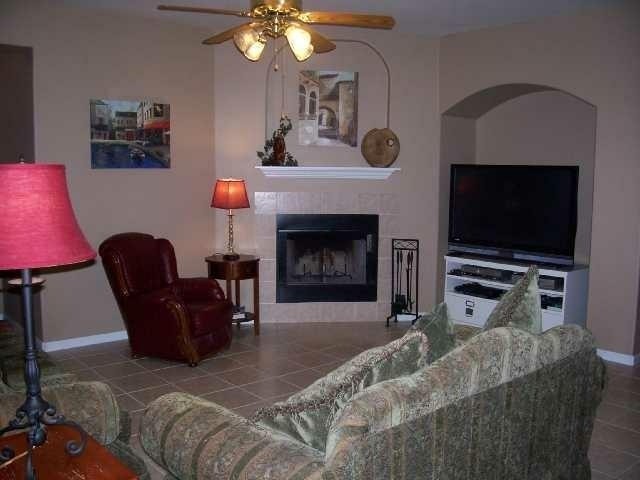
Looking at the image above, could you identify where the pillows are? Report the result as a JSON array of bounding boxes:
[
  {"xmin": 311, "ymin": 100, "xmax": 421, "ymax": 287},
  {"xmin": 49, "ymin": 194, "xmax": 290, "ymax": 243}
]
[
  {"xmin": 249, "ymin": 330, "xmax": 428, "ymax": 453},
  {"xmin": 478, "ymin": 264, "xmax": 541, "ymax": 338},
  {"xmin": 404, "ymin": 301, "xmax": 451, "ymax": 368}
]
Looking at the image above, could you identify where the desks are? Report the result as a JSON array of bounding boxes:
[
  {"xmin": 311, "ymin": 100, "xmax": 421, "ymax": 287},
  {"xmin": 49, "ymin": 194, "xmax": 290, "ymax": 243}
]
[
  {"xmin": 203, "ymin": 255, "xmax": 261, "ymax": 338},
  {"xmin": 0, "ymin": 420, "xmax": 142, "ymax": 480}
]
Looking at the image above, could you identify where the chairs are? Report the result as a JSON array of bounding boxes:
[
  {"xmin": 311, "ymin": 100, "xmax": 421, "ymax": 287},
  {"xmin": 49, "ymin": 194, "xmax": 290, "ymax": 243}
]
[{"xmin": 98, "ymin": 232, "xmax": 234, "ymax": 367}]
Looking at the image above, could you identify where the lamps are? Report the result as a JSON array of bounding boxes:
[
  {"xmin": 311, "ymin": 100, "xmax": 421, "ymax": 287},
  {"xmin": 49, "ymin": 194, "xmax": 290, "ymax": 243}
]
[
  {"xmin": 210, "ymin": 177, "xmax": 251, "ymax": 261},
  {"xmin": 0, "ymin": 153, "xmax": 98, "ymax": 480},
  {"xmin": 234, "ymin": 15, "xmax": 314, "ymax": 62}
]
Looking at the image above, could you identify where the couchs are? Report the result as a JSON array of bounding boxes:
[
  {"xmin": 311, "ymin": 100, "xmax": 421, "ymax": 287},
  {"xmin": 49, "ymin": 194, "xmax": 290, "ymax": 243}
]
[
  {"xmin": 138, "ymin": 322, "xmax": 610, "ymax": 480},
  {"xmin": 0, "ymin": 319, "xmax": 151, "ymax": 480}
]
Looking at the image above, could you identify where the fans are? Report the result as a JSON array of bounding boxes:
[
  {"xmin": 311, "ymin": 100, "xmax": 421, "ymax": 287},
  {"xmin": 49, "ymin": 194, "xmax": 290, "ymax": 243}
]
[{"xmin": 156, "ymin": 0, "xmax": 397, "ymax": 53}]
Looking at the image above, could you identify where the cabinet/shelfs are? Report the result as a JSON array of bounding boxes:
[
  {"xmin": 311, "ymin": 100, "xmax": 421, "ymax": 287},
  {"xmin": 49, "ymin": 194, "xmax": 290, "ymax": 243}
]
[{"xmin": 442, "ymin": 250, "xmax": 589, "ymax": 334}]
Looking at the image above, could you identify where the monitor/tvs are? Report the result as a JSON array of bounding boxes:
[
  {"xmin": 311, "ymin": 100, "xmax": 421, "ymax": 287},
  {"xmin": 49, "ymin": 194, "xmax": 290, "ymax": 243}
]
[{"xmin": 446, "ymin": 163, "xmax": 578, "ymax": 266}]
